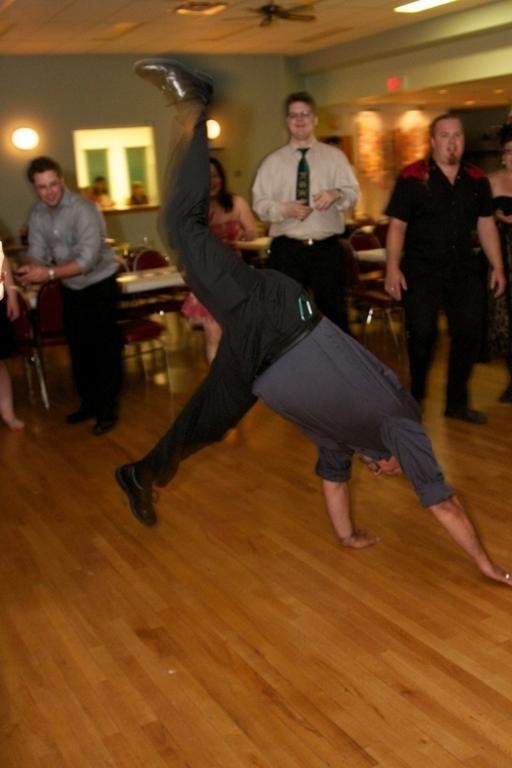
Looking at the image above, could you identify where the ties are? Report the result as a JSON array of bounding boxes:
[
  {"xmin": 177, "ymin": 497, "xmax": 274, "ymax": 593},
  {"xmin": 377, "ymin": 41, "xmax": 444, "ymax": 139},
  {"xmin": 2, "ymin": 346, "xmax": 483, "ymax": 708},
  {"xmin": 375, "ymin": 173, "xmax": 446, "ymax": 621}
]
[{"xmin": 296, "ymin": 148, "xmax": 311, "ymax": 221}]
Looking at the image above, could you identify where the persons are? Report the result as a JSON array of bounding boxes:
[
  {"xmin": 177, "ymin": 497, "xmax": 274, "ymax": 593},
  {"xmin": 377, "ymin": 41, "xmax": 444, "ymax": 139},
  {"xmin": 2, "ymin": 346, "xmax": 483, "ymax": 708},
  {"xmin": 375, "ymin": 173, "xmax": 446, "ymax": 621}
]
[
  {"xmin": 114, "ymin": 58, "xmax": 512, "ymax": 584},
  {"xmin": 0, "ymin": 239, "xmax": 28, "ymax": 429},
  {"xmin": 480, "ymin": 126, "xmax": 512, "ymax": 349},
  {"xmin": 130, "ymin": 179, "xmax": 147, "ymax": 205},
  {"xmin": 93, "ymin": 177, "xmax": 114, "ymax": 209},
  {"xmin": 252, "ymin": 94, "xmax": 361, "ymax": 335},
  {"xmin": 383, "ymin": 115, "xmax": 505, "ymax": 424},
  {"xmin": 180, "ymin": 156, "xmax": 260, "ymax": 372},
  {"xmin": 14, "ymin": 156, "xmax": 121, "ymax": 435}
]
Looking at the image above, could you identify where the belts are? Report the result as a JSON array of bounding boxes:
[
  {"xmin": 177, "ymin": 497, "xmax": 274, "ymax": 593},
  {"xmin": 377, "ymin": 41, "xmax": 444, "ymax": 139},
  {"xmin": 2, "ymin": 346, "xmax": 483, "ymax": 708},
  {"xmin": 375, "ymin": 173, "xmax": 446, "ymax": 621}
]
[
  {"xmin": 245, "ymin": 313, "xmax": 324, "ymax": 386},
  {"xmin": 280, "ymin": 234, "xmax": 345, "ymax": 246}
]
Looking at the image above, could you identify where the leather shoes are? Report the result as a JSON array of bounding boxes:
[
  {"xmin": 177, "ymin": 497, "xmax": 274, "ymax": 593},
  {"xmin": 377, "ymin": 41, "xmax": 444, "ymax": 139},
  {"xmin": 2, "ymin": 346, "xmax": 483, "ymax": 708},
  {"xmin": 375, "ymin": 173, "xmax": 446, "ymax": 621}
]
[
  {"xmin": 115, "ymin": 464, "xmax": 158, "ymax": 527},
  {"xmin": 67, "ymin": 410, "xmax": 94, "ymax": 423},
  {"xmin": 134, "ymin": 58, "xmax": 215, "ymax": 107},
  {"xmin": 444, "ymin": 403, "xmax": 487, "ymax": 424},
  {"xmin": 94, "ymin": 421, "xmax": 118, "ymax": 435}
]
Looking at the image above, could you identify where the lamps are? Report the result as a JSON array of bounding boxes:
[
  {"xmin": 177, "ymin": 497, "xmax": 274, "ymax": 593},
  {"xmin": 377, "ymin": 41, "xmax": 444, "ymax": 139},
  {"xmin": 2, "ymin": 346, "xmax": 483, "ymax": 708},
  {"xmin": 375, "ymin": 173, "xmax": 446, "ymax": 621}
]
[
  {"xmin": 11, "ymin": 128, "xmax": 39, "ymax": 150},
  {"xmin": 206, "ymin": 119, "xmax": 221, "ymax": 140}
]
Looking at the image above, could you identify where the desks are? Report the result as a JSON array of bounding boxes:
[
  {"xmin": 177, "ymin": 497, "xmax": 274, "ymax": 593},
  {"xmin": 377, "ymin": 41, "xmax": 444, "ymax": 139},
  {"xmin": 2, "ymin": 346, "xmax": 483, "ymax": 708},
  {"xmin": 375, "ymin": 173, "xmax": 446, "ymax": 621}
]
[{"xmin": 117, "ymin": 263, "xmax": 192, "ymax": 319}]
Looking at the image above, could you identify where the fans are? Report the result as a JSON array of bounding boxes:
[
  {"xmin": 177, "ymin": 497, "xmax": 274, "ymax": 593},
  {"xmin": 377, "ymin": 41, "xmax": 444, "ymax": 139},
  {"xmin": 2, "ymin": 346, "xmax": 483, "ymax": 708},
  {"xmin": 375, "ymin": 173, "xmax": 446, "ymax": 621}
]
[{"xmin": 221, "ymin": 0, "xmax": 315, "ymax": 28}]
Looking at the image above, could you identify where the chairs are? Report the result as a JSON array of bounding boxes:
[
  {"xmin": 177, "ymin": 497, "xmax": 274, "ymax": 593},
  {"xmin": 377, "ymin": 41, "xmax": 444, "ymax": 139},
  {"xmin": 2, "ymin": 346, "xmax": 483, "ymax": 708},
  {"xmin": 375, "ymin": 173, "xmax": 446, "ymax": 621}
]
[
  {"xmin": 5, "ymin": 285, "xmax": 33, "ymax": 411},
  {"xmin": 349, "ymin": 233, "xmax": 405, "ymax": 352},
  {"xmin": 108, "ymin": 258, "xmax": 161, "ymax": 387},
  {"xmin": 132, "ymin": 249, "xmax": 168, "ymax": 272},
  {"xmin": 29, "ymin": 282, "xmax": 67, "ymax": 410}
]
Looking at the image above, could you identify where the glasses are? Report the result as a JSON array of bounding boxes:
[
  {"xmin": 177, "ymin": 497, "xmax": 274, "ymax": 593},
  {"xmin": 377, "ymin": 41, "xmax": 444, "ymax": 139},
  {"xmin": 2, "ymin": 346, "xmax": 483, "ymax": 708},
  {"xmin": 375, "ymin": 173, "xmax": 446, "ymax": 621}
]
[
  {"xmin": 32, "ymin": 181, "xmax": 61, "ymax": 188},
  {"xmin": 287, "ymin": 111, "xmax": 313, "ymax": 117}
]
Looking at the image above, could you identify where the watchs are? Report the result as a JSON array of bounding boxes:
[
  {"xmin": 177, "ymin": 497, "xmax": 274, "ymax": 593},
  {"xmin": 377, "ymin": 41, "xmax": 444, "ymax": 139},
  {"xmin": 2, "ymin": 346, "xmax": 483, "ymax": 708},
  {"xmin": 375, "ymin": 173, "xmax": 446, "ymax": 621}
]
[{"xmin": 47, "ymin": 265, "xmax": 55, "ymax": 280}]
